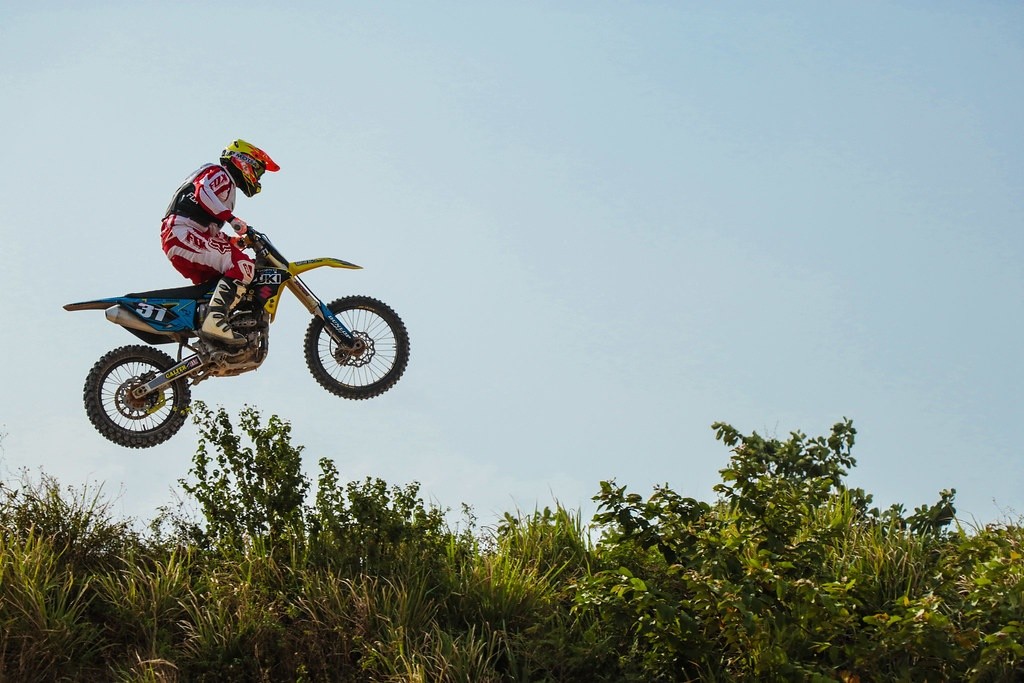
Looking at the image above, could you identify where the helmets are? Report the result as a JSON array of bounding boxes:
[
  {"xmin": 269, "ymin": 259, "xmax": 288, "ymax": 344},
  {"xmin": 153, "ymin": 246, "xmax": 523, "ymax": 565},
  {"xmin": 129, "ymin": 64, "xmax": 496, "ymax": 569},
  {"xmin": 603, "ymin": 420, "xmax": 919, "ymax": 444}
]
[{"xmin": 219, "ymin": 137, "xmax": 280, "ymax": 197}]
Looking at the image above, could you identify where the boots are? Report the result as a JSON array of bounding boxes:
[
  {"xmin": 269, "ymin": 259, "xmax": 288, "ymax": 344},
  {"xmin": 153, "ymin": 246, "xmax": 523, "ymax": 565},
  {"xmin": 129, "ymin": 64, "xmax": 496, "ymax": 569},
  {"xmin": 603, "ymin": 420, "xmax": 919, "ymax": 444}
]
[{"xmin": 202, "ymin": 277, "xmax": 249, "ymax": 346}]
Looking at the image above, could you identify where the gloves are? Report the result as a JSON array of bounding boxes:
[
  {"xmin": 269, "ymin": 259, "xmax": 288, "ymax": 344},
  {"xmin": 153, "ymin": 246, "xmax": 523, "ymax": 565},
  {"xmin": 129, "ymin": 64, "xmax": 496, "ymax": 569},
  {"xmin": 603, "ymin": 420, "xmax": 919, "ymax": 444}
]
[
  {"xmin": 230, "ymin": 237, "xmax": 247, "ymax": 252},
  {"xmin": 230, "ymin": 217, "xmax": 247, "ymax": 235}
]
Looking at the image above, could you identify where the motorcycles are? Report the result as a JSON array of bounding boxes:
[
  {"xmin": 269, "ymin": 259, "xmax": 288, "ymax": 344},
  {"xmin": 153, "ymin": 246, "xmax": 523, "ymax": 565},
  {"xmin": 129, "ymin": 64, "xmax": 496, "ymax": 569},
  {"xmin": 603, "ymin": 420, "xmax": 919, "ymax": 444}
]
[{"xmin": 61, "ymin": 221, "xmax": 413, "ymax": 451}]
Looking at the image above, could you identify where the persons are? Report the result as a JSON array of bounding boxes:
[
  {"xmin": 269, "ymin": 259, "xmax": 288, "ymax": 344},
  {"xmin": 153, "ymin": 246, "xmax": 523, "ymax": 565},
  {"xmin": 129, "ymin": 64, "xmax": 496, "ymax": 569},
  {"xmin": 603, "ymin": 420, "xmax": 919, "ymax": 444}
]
[{"xmin": 160, "ymin": 138, "xmax": 280, "ymax": 345}]
